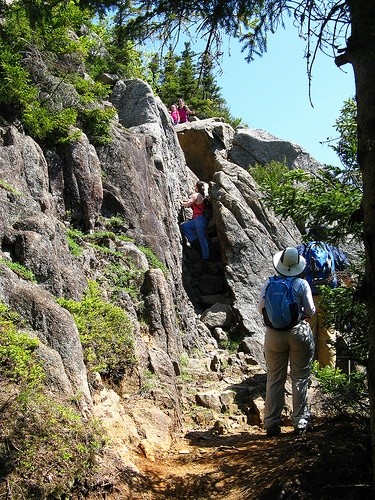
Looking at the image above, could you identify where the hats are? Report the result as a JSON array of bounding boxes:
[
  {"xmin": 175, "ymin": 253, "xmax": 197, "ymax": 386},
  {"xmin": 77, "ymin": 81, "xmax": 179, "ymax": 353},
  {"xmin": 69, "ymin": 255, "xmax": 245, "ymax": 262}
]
[{"xmin": 272, "ymin": 246, "xmax": 308, "ymax": 277}]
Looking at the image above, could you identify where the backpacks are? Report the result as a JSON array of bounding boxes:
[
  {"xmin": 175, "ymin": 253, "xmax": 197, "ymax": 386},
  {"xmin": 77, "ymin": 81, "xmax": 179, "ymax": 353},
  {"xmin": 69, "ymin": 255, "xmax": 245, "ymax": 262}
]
[
  {"xmin": 193, "ymin": 195, "xmax": 213, "ymax": 220},
  {"xmin": 261, "ymin": 277, "xmax": 304, "ymax": 331},
  {"xmin": 303, "ymin": 240, "xmax": 335, "ymax": 296}
]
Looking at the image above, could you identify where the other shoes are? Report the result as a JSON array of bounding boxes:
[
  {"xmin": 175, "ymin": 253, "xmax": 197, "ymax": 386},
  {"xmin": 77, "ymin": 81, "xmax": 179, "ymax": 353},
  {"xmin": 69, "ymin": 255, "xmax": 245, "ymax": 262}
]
[
  {"xmin": 200, "ymin": 257, "xmax": 211, "ymax": 261},
  {"xmin": 266, "ymin": 425, "xmax": 281, "ymax": 437},
  {"xmin": 190, "ymin": 238, "xmax": 199, "ymax": 250},
  {"xmin": 293, "ymin": 428, "xmax": 308, "ymax": 435}
]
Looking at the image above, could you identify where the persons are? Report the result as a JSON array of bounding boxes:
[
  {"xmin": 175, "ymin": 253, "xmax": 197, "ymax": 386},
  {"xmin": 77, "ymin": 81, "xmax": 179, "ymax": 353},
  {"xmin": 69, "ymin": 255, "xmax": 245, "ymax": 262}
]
[
  {"xmin": 296, "ymin": 227, "xmax": 354, "ymax": 388},
  {"xmin": 178, "ymin": 99, "xmax": 195, "ymax": 123},
  {"xmin": 179, "ymin": 181, "xmax": 209, "ymax": 263},
  {"xmin": 168, "ymin": 105, "xmax": 180, "ymax": 124},
  {"xmin": 258, "ymin": 247, "xmax": 316, "ymax": 436}
]
